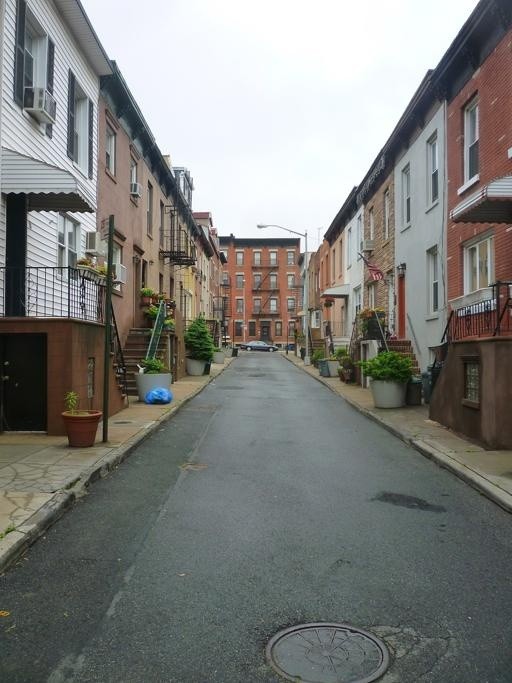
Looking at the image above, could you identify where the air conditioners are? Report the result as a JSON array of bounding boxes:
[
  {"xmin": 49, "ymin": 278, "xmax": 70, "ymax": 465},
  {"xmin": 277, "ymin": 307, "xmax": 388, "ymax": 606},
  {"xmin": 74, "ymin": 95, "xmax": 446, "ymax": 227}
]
[
  {"xmin": 358, "ymin": 238, "xmax": 376, "ymax": 253},
  {"xmin": 129, "ymin": 181, "xmax": 144, "ymax": 199},
  {"xmin": 85, "ymin": 230, "xmax": 106, "ymax": 257},
  {"xmin": 112, "ymin": 262, "xmax": 128, "ymax": 285},
  {"xmin": 21, "ymin": 84, "xmax": 58, "ymax": 126}
]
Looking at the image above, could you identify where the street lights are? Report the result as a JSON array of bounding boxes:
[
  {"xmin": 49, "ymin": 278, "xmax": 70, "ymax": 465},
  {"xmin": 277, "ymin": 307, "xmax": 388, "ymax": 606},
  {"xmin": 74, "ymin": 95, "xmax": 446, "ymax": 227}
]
[{"xmin": 256, "ymin": 222, "xmax": 312, "ymax": 368}]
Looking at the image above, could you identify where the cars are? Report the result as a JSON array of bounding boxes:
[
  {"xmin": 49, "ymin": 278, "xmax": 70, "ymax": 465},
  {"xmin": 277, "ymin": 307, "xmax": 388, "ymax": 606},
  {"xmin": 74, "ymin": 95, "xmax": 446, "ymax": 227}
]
[
  {"xmin": 240, "ymin": 339, "xmax": 279, "ymax": 351},
  {"xmin": 285, "ymin": 343, "xmax": 295, "ymax": 350}
]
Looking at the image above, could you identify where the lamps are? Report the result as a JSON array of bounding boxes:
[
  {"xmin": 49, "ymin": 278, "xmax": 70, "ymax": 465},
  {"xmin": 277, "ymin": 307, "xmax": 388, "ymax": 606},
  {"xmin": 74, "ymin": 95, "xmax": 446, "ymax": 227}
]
[{"xmin": 396, "ymin": 261, "xmax": 407, "ymax": 279}]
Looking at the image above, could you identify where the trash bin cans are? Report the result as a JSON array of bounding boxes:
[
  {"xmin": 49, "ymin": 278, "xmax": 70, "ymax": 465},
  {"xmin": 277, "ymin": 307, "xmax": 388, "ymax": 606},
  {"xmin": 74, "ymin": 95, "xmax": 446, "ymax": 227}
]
[
  {"xmin": 232, "ymin": 348, "xmax": 238, "ymax": 357},
  {"xmin": 406, "ymin": 381, "xmax": 423, "ymax": 405},
  {"xmin": 300, "ymin": 346, "xmax": 306, "ymax": 360}
]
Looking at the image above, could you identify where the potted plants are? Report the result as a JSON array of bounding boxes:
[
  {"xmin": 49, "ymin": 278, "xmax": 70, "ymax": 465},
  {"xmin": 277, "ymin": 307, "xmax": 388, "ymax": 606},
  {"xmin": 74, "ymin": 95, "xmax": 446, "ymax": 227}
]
[
  {"xmin": 293, "ymin": 307, "xmax": 414, "ymax": 410},
  {"xmin": 60, "ymin": 388, "xmax": 105, "ymax": 449},
  {"xmin": 132, "ymin": 284, "xmax": 225, "ymax": 406},
  {"xmin": 76, "ymin": 256, "xmax": 115, "ymax": 287}
]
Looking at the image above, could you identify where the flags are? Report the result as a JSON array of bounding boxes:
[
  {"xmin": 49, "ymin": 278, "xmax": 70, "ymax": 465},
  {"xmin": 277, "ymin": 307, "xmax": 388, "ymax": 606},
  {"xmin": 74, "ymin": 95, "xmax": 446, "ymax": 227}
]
[{"xmin": 360, "ymin": 255, "xmax": 384, "ymax": 281}]
